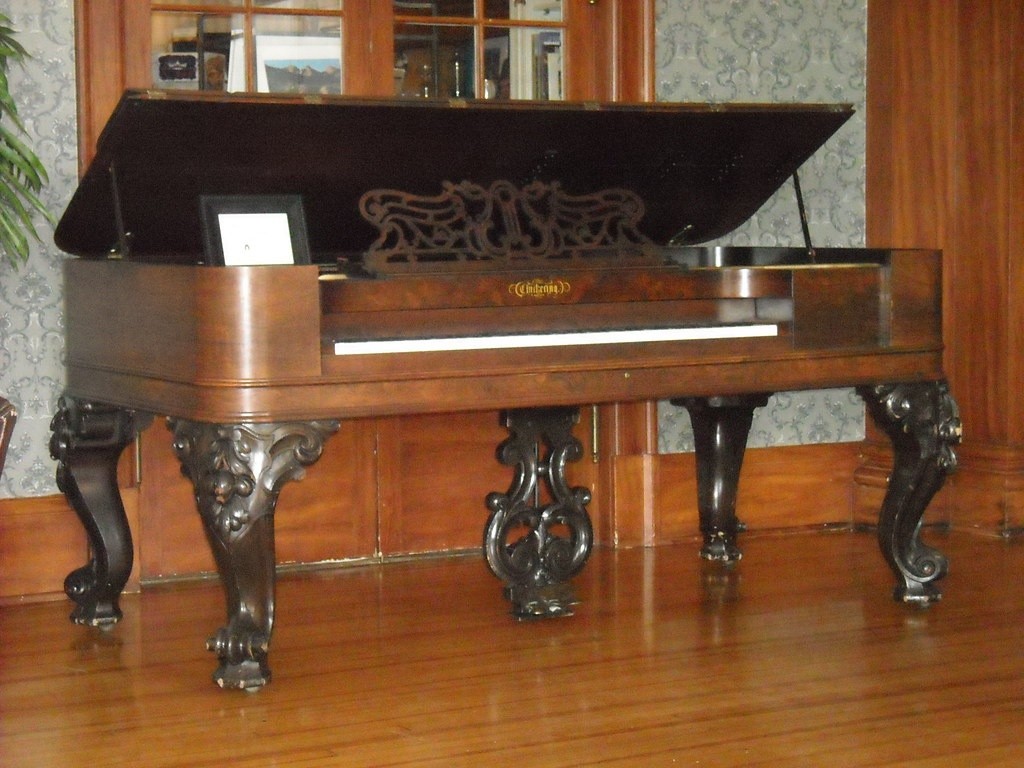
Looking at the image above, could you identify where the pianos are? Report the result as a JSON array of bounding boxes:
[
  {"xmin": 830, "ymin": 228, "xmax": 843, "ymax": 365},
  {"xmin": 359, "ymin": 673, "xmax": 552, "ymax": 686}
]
[{"xmin": 50, "ymin": 90, "xmax": 961, "ymax": 693}]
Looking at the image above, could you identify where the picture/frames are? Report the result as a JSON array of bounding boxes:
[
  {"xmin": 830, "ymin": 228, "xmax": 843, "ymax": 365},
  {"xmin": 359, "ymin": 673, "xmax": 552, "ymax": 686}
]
[{"xmin": 195, "ymin": 191, "xmax": 310, "ymax": 267}]
